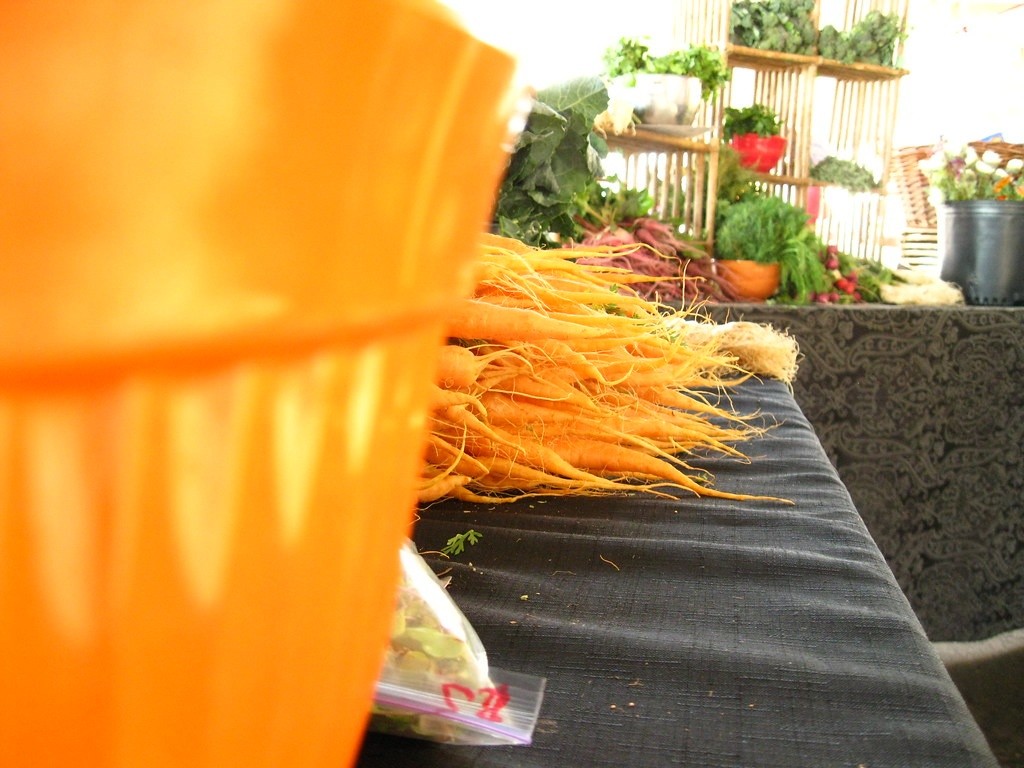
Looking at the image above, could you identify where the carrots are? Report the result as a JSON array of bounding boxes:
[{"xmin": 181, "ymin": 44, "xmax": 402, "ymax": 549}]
[{"xmin": 407, "ymin": 231, "xmax": 799, "ymax": 507}]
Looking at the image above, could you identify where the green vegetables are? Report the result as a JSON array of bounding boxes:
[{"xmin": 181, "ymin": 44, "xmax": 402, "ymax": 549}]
[{"xmin": 494, "ymin": 0, "xmax": 902, "ymax": 312}]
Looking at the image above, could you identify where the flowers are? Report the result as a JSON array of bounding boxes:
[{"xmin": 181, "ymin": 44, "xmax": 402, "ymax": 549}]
[{"xmin": 917, "ymin": 135, "xmax": 1024, "ymax": 200}]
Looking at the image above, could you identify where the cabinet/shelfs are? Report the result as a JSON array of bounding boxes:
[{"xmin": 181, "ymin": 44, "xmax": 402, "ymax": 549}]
[{"xmin": 588, "ymin": 0, "xmax": 910, "ymax": 269}]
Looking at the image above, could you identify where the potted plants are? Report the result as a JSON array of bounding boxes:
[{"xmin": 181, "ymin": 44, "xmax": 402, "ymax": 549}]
[
  {"xmin": 723, "ymin": 103, "xmax": 786, "ymax": 172},
  {"xmin": 601, "ymin": 33, "xmax": 732, "ymax": 126}
]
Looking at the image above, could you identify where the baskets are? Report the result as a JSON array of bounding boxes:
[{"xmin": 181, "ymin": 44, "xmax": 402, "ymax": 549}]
[{"xmin": 892, "ymin": 141, "xmax": 1024, "ymax": 229}]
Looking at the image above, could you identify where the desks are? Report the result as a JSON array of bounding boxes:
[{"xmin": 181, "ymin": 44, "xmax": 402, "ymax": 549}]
[
  {"xmin": 355, "ymin": 374, "xmax": 999, "ymax": 768},
  {"xmin": 660, "ymin": 300, "xmax": 1024, "ymax": 641}
]
